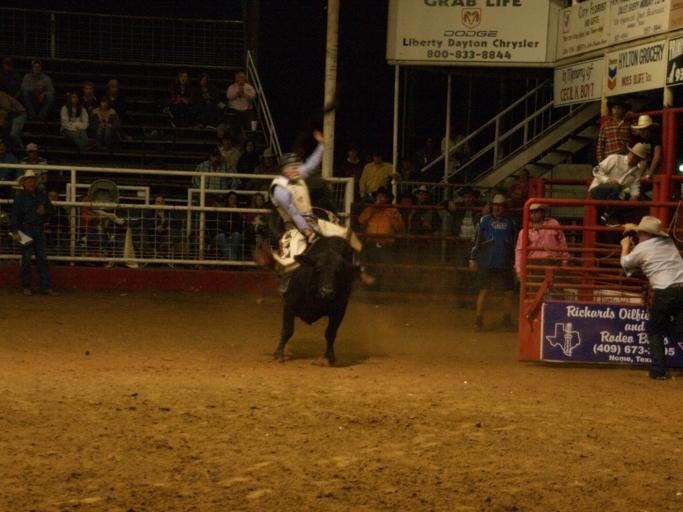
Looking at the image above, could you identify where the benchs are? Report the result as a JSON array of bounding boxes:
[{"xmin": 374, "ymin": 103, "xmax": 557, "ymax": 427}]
[{"xmin": 0, "ymin": 62, "xmax": 272, "ymax": 256}]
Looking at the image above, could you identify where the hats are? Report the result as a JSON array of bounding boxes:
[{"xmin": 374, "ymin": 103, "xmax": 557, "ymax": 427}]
[
  {"xmin": 633, "ymin": 216, "xmax": 669, "ymax": 237},
  {"xmin": 18, "ymin": 169, "xmax": 41, "ymax": 186},
  {"xmin": 630, "ymin": 115, "xmax": 658, "ymax": 128},
  {"xmin": 488, "ymin": 194, "xmax": 512, "ymax": 207},
  {"xmin": 530, "ymin": 202, "xmax": 548, "ymax": 211},
  {"xmin": 626, "ymin": 143, "xmax": 650, "ymax": 161}
]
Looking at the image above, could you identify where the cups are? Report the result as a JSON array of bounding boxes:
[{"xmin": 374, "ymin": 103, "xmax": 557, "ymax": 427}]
[{"xmin": 251, "ymin": 120, "xmax": 257, "ymax": 132}]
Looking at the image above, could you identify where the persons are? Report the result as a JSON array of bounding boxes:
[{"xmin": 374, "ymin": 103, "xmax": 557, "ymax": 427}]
[
  {"xmin": 342, "ymin": 125, "xmax": 569, "ymax": 333},
  {"xmin": 588, "ymin": 98, "xmax": 683, "ymax": 379},
  {"xmin": 1, "ymin": 58, "xmax": 283, "ymax": 296},
  {"xmin": 268, "ymin": 131, "xmax": 376, "ymax": 293}
]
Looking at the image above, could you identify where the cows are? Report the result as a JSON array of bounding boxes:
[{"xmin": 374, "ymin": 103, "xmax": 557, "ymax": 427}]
[{"xmin": 257, "ymin": 187, "xmax": 363, "ymax": 366}]
